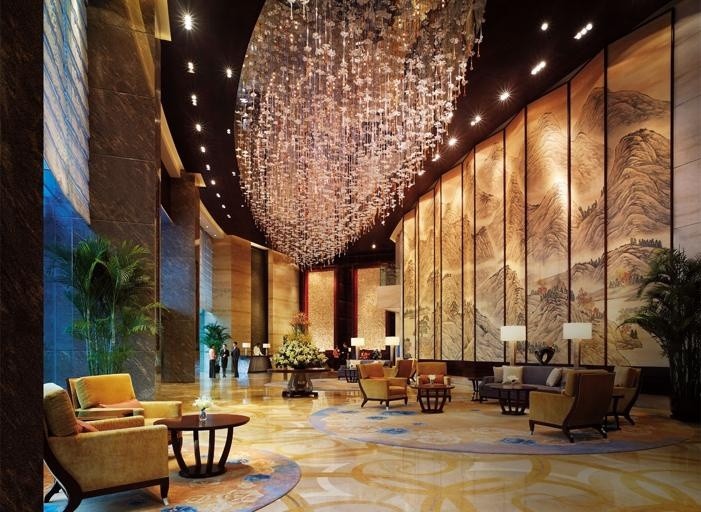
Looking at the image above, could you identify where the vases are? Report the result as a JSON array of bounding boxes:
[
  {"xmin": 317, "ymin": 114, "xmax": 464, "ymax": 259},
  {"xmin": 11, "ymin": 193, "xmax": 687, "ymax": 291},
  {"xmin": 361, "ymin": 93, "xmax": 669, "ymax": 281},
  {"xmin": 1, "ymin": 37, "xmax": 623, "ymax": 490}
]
[
  {"xmin": 285, "ymin": 365, "xmax": 313, "ymax": 393},
  {"xmin": 198, "ymin": 409, "xmax": 209, "ymax": 422}
]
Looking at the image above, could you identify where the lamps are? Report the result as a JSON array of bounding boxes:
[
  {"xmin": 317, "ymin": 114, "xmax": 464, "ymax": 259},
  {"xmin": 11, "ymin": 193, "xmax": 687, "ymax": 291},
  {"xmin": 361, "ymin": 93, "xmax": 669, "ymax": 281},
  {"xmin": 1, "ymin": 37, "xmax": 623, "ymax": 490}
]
[
  {"xmin": 263, "ymin": 343, "xmax": 271, "ymax": 355},
  {"xmin": 242, "ymin": 342, "xmax": 251, "ymax": 355},
  {"xmin": 384, "ymin": 337, "xmax": 399, "ymax": 359},
  {"xmin": 234, "ymin": 2, "xmax": 488, "ymax": 272},
  {"xmin": 351, "ymin": 337, "xmax": 364, "ymax": 360},
  {"xmin": 500, "ymin": 324, "xmax": 526, "ymax": 364},
  {"xmin": 561, "ymin": 322, "xmax": 592, "ymax": 366}
]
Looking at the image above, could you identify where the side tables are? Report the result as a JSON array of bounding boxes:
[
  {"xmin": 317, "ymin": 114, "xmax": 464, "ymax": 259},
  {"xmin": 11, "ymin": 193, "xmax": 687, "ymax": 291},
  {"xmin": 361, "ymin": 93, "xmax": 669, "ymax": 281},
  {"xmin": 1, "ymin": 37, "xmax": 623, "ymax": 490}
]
[
  {"xmin": 468, "ymin": 376, "xmax": 487, "ymax": 402},
  {"xmin": 600, "ymin": 392, "xmax": 625, "ymax": 434}
]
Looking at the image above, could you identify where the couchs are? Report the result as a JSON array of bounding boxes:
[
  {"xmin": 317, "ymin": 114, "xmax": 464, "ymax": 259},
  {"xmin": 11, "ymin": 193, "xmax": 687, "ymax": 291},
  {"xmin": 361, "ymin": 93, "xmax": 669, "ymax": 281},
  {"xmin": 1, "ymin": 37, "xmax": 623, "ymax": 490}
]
[{"xmin": 481, "ymin": 364, "xmax": 588, "ymax": 409}]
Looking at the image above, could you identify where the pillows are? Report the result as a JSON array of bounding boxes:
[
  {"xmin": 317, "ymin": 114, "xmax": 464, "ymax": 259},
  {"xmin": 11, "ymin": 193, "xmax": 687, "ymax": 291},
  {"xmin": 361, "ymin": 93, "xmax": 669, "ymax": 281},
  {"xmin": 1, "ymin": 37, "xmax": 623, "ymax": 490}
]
[
  {"xmin": 560, "ymin": 365, "xmax": 575, "ymax": 388},
  {"xmin": 97, "ymin": 398, "xmax": 146, "ymax": 417},
  {"xmin": 76, "ymin": 416, "xmax": 102, "ymax": 434},
  {"xmin": 546, "ymin": 366, "xmax": 563, "ymax": 387},
  {"xmin": 502, "ymin": 365, "xmax": 525, "ymax": 385},
  {"xmin": 491, "ymin": 365, "xmax": 504, "ymax": 382}
]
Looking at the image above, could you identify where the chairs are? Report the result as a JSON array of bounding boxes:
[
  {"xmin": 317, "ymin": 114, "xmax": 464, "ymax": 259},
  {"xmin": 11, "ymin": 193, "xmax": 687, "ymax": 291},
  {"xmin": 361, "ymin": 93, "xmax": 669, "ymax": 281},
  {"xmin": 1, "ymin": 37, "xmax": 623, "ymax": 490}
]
[
  {"xmin": 603, "ymin": 365, "xmax": 645, "ymax": 425},
  {"xmin": 65, "ymin": 372, "xmax": 186, "ymax": 457},
  {"xmin": 525, "ymin": 367, "xmax": 617, "ymax": 442},
  {"xmin": 332, "ymin": 347, "xmax": 458, "ymax": 414},
  {"xmin": 40, "ymin": 381, "xmax": 170, "ymax": 512}
]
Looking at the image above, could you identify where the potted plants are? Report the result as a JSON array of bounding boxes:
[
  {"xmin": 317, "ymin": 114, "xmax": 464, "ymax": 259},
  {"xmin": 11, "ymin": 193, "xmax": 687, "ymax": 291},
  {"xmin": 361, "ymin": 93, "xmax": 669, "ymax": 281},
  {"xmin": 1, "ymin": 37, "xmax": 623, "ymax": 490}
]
[
  {"xmin": 201, "ymin": 320, "xmax": 233, "ymax": 373},
  {"xmin": 623, "ymin": 242, "xmax": 701, "ymax": 423}
]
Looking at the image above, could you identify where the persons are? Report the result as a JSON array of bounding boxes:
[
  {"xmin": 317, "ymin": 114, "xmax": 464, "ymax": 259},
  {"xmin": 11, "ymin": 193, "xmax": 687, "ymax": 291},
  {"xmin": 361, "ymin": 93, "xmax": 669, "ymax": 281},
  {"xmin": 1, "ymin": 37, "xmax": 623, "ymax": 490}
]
[
  {"xmin": 220, "ymin": 344, "xmax": 229, "ymax": 377},
  {"xmin": 209, "ymin": 344, "xmax": 217, "ymax": 378},
  {"xmin": 231, "ymin": 342, "xmax": 240, "ymax": 377},
  {"xmin": 333, "ymin": 342, "xmax": 380, "ymax": 371},
  {"xmin": 252, "ymin": 343, "xmax": 264, "ymax": 356}
]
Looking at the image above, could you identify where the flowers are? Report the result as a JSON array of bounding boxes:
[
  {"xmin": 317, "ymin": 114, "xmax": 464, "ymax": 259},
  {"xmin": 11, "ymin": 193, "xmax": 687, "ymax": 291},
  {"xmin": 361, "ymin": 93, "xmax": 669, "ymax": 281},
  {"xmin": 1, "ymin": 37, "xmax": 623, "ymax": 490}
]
[
  {"xmin": 193, "ymin": 395, "xmax": 214, "ymax": 410},
  {"xmin": 266, "ymin": 312, "xmax": 328, "ymax": 367}
]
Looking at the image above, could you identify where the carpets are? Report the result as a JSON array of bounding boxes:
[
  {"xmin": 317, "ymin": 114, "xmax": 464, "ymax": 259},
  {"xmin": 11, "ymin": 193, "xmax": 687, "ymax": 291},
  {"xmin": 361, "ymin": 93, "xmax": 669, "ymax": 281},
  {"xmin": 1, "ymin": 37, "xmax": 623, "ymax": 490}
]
[
  {"xmin": 39, "ymin": 437, "xmax": 301, "ymax": 511},
  {"xmin": 264, "ymin": 375, "xmax": 361, "ymax": 392},
  {"xmin": 307, "ymin": 390, "xmax": 695, "ymax": 457}
]
